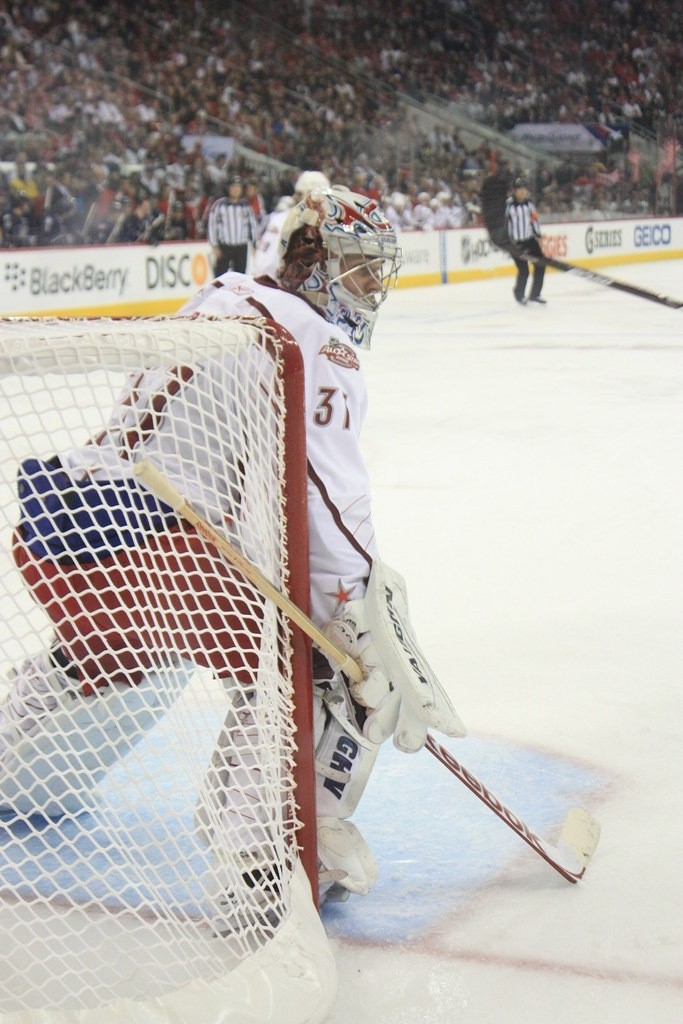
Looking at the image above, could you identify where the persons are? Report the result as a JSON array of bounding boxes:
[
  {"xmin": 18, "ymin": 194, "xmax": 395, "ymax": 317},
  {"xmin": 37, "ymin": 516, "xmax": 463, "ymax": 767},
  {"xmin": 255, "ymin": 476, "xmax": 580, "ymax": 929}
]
[
  {"xmin": 255, "ymin": 171, "xmax": 330, "ymax": 285},
  {"xmin": 504, "ymin": 178, "xmax": 546, "ymax": 304},
  {"xmin": 0, "ymin": 185, "xmax": 429, "ymax": 937},
  {"xmin": 208, "ymin": 175, "xmax": 259, "ymax": 280},
  {"xmin": 0, "ymin": 0, "xmax": 683, "ymax": 249}
]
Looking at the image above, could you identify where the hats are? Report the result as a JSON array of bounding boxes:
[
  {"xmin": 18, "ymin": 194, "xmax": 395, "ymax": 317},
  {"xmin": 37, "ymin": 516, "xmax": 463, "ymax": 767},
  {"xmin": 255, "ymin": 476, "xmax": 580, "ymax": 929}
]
[{"xmin": 391, "ymin": 190, "xmax": 451, "ymax": 207}]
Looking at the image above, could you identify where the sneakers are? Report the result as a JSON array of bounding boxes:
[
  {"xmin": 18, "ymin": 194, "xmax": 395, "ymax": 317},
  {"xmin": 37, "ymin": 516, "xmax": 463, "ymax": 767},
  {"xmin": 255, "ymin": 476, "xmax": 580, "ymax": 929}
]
[
  {"xmin": 513, "ymin": 289, "xmax": 528, "ymax": 308},
  {"xmin": 528, "ymin": 295, "xmax": 546, "ymax": 307}
]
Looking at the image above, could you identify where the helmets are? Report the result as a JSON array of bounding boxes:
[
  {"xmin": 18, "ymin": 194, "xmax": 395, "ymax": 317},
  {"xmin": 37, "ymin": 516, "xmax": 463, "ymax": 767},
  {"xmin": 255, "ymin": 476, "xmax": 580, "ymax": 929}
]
[
  {"xmin": 172, "ymin": 201, "xmax": 184, "ymax": 212},
  {"xmin": 12, "ymin": 190, "xmax": 26, "ymax": 203},
  {"xmin": 513, "ymin": 178, "xmax": 526, "ymax": 188},
  {"xmin": 227, "ymin": 175, "xmax": 241, "ymax": 183},
  {"xmin": 110, "ymin": 196, "xmax": 130, "ymax": 211},
  {"xmin": 277, "ymin": 188, "xmax": 404, "ymax": 352},
  {"xmin": 294, "ymin": 171, "xmax": 330, "ymax": 193}
]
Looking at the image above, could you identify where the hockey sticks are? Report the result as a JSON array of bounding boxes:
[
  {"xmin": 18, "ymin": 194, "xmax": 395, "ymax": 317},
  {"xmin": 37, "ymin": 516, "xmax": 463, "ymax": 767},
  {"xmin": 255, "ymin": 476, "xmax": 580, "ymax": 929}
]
[
  {"xmin": 481, "ymin": 176, "xmax": 683, "ymax": 313},
  {"xmin": 130, "ymin": 460, "xmax": 603, "ymax": 884}
]
